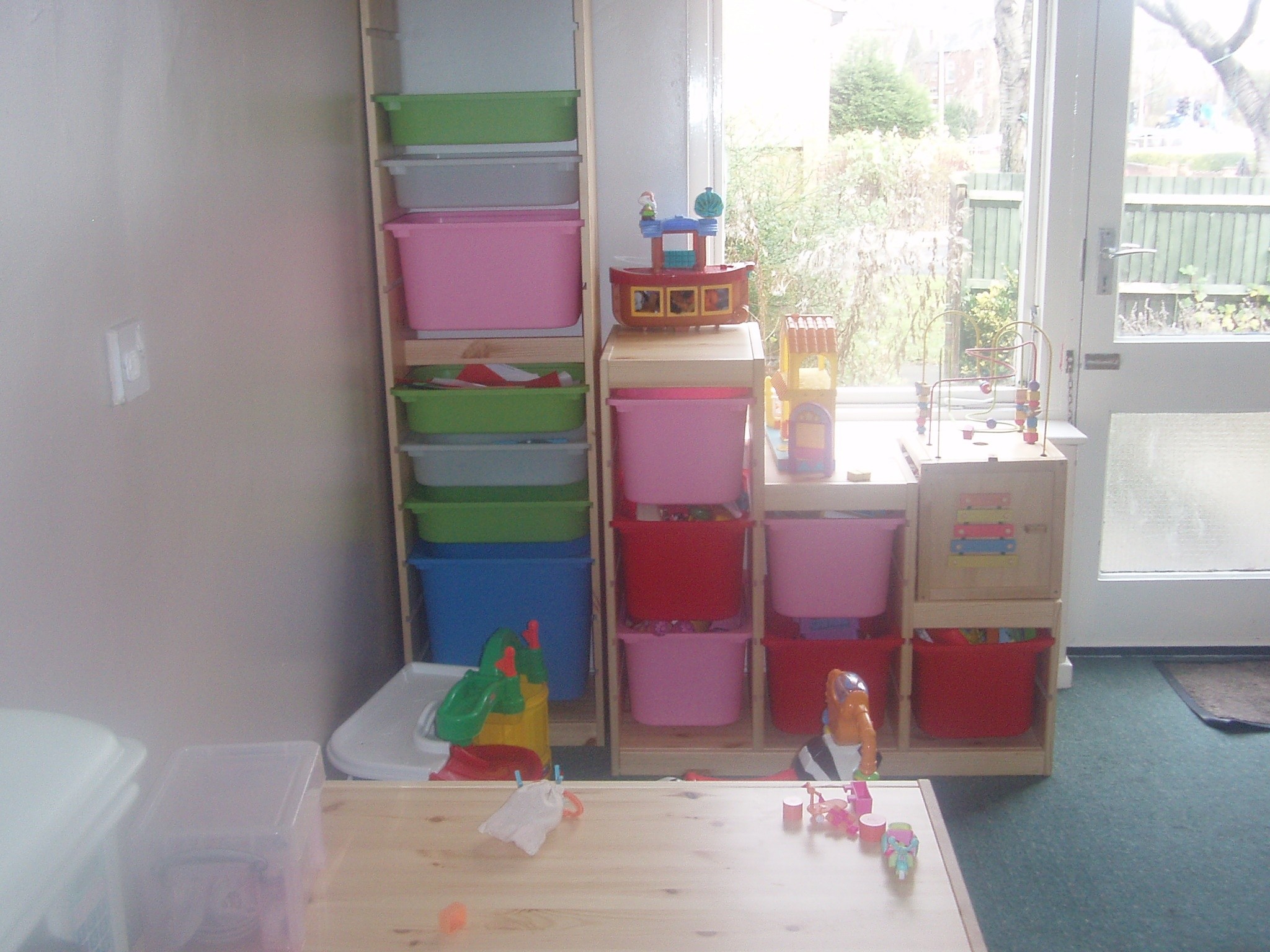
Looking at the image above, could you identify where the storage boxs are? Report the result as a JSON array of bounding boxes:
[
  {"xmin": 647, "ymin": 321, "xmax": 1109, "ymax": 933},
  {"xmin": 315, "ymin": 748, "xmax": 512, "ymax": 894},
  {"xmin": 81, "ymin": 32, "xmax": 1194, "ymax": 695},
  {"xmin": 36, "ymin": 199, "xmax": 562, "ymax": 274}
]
[{"xmin": 0, "ymin": 90, "xmax": 1055, "ymax": 952}]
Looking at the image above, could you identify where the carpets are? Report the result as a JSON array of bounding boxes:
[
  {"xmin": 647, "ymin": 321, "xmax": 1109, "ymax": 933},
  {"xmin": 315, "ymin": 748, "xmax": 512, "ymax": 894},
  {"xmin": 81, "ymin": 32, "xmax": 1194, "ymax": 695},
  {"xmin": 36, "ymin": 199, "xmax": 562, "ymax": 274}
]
[{"xmin": 1152, "ymin": 658, "xmax": 1270, "ymax": 728}]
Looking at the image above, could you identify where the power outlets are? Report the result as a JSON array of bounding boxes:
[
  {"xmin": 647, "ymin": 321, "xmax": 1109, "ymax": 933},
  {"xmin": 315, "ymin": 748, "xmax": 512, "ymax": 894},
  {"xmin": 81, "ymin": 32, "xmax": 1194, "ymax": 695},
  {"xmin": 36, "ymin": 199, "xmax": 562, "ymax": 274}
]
[{"xmin": 106, "ymin": 320, "xmax": 149, "ymax": 406}]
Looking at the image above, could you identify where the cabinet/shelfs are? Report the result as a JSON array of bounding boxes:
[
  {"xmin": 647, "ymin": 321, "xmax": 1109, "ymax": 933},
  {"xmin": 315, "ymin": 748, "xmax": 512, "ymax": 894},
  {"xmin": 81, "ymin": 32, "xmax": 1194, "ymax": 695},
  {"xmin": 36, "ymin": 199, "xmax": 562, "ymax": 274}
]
[
  {"xmin": 601, "ymin": 323, "xmax": 1068, "ymax": 778},
  {"xmin": 359, "ymin": 0, "xmax": 603, "ymax": 747}
]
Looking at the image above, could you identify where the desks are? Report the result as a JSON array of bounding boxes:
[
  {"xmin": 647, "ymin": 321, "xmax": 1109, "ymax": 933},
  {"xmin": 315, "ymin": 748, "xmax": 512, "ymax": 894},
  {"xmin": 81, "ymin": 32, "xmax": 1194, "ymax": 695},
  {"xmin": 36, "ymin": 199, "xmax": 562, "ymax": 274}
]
[{"xmin": 312, "ymin": 776, "xmax": 987, "ymax": 952}]
